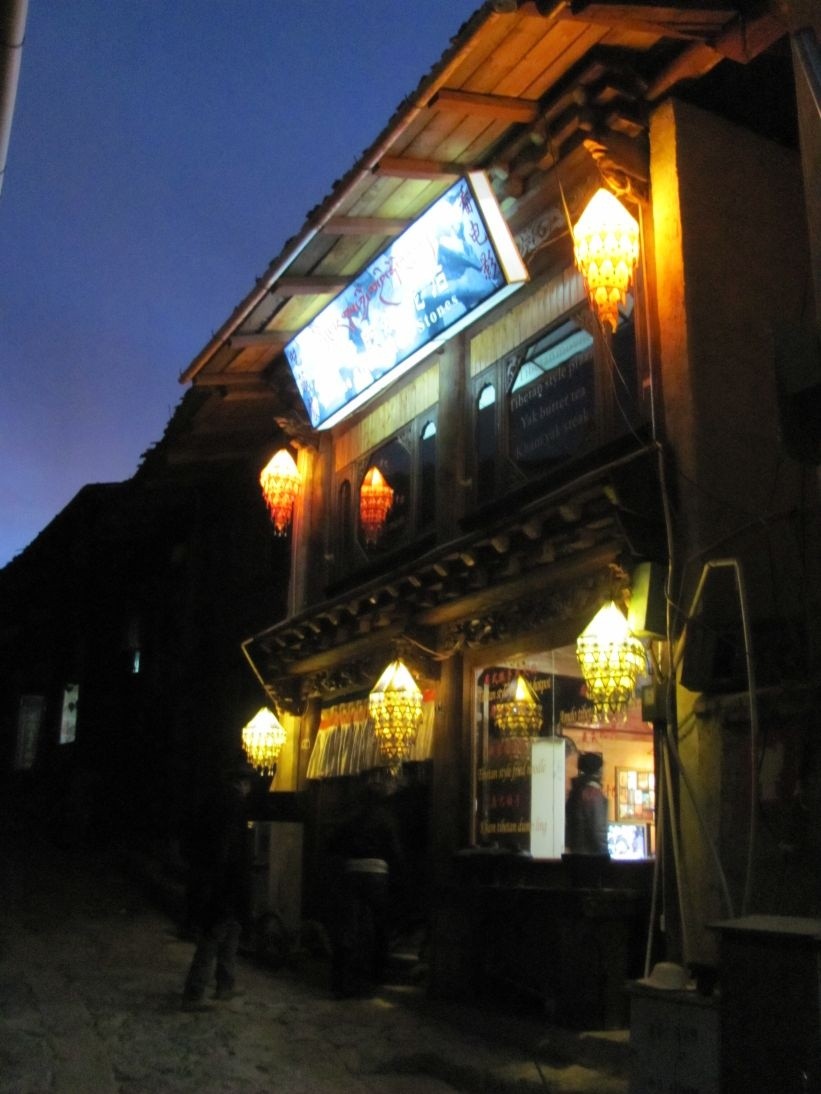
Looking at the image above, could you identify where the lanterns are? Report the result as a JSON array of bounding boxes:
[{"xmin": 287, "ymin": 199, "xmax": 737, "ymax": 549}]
[
  {"xmin": 491, "ymin": 672, "xmax": 544, "ymax": 764},
  {"xmin": 572, "ymin": 187, "xmax": 639, "ymax": 336},
  {"xmin": 358, "ymin": 464, "xmax": 394, "ymax": 546},
  {"xmin": 258, "ymin": 447, "xmax": 301, "ymax": 537},
  {"xmin": 368, "ymin": 660, "xmax": 423, "ymax": 784},
  {"xmin": 575, "ymin": 600, "xmax": 649, "ymax": 726},
  {"xmin": 242, "ymin": 706, "xmax": 287, "ymax": 777}
]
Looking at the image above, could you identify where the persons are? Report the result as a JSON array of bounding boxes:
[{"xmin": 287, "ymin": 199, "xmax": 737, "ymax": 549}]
[
  {"xmin": 564, "ymin": 752, "xmax": 609, "ymax": 853},
  {"xmin": 179, "ymin": 768, "xmax": 255, "ymax": 1012}
]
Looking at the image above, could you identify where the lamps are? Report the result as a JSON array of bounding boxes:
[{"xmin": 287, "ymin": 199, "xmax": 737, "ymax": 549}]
[
  {"xmin": 369, "ymin": 648, "xmax": 424, "ymax": 778},
  {"xmin": 572, "ymin": 188, "xmax": 640, "ymax": 334},
  {"xmin": 494, "ymin": 668, "xmax": 542, "ymax": 777},
  {"xmin": 242, "ymin": 689, "xmax": 285, "ymax": 777},
  {"xmin": 575, "ymin": 568, "xmax": 646, "ymax": 724},
  {"xmin": 360, "ymin": 465, "xmax": 394, "ymax": 544},
  {"xmin": 259, "ymin": 448, "xmax": 302, "ymax": 536}
]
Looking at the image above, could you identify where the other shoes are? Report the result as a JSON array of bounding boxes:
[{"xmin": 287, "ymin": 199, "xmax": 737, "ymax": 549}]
[
  {"xmin": 218, "ymin": 982, "xmax": 244, "ymax": 1000},
  {"xmin": 184, "ymin": 997, "xmax": 214, "ymax": 1011}
]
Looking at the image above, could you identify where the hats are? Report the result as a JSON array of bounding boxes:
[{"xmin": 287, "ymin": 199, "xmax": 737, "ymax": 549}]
[
  {"xmin": 221, "ymin": 760, "xmax": 257, "ymax": 777},
  {"xmin": 577, "ymin": 753, "xmax": 602, "ymax": 774}
]
